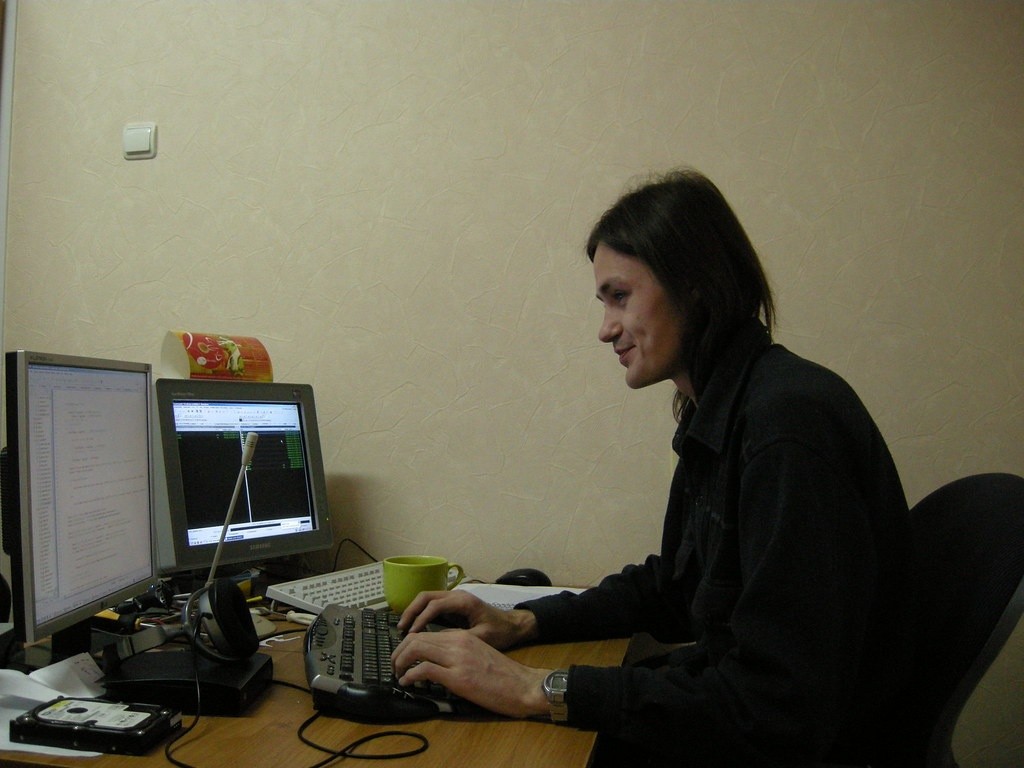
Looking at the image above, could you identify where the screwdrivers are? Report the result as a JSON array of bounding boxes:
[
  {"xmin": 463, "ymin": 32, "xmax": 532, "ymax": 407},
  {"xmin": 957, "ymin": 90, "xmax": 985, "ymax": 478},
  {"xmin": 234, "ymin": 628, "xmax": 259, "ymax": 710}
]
[{"xmin": 258, "ymin": 605, "xmax": 318, "ymax": 626}]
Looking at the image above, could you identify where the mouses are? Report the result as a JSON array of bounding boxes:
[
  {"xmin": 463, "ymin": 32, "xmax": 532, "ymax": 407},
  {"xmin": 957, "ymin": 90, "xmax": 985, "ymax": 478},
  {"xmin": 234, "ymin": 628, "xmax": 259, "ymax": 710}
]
[{"xmin": 497, "ymin": 568, "xmax": 552, "ymax": 587}]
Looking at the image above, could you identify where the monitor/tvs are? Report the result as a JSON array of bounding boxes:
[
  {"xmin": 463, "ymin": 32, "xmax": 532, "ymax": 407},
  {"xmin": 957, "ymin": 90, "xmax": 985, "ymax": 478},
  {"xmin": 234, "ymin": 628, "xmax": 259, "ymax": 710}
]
[
  {"xmin": 153, "ymin": 377, "xmax": 334, "ymax": 614},
  {"xmin": 0, "ymin": 350, "xmax": 167, "ymax": 672}
]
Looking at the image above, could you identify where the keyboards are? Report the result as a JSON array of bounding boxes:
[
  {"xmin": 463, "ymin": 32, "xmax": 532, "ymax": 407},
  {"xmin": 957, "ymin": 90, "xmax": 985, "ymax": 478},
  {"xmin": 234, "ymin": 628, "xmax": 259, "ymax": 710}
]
[
  {"xmin": 265, "ymin": 561, "xmax": 458, "ymax": 614},
  {"xmin": 305, "ymin": 603, "xmax": 472, "ymax": 722}
]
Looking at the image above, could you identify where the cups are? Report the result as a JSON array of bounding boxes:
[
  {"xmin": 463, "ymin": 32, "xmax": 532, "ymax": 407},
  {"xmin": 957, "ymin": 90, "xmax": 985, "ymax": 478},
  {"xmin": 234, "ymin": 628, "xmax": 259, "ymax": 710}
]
[{"xmin": 383, "ymin": 555, "xmax": 464, "ymax": 615}]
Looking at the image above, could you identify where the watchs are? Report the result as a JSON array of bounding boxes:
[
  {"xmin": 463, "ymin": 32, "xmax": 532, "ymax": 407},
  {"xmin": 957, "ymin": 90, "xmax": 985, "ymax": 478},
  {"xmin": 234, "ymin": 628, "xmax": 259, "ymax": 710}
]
[{"xmin": 543, "ymin": 669, "xmax": 568, "ymax": 721}]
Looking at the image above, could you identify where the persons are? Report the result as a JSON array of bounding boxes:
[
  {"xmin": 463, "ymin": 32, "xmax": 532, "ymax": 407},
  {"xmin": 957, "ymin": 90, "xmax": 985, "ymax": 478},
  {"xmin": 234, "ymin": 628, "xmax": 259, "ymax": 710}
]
[{"xmin": 392, "ymin": 172, "xmax": 909, "ymax": 768}]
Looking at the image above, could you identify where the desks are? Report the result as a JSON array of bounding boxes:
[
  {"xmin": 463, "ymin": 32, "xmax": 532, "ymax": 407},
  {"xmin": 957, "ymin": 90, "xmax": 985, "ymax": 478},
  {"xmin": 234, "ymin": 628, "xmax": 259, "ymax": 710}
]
[{"xmin": 0, "ymin": 582, "xmax": 632, "ymax": 768}]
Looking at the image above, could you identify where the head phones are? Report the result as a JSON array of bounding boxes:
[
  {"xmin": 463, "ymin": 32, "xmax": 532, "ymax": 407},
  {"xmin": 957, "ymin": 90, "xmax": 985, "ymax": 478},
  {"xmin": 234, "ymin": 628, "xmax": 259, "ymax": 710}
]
[{"xmin": 93, "ymin": 578, "xmax": 260, "ymax": 668}]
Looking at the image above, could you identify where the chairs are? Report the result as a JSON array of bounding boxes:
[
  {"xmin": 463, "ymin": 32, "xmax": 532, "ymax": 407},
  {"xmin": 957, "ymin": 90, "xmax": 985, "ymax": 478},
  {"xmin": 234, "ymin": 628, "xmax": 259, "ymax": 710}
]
[{"xmin": 856, "ymin": 472, "xmax": 1024, "ymax": 768}]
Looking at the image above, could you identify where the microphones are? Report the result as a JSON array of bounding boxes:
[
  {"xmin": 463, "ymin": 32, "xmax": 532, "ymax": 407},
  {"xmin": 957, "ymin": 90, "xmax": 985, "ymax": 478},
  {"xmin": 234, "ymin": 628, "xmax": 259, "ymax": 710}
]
[{"xmin": 106, "ymin": 433, "xmax": 273, "ymax": 717}]
